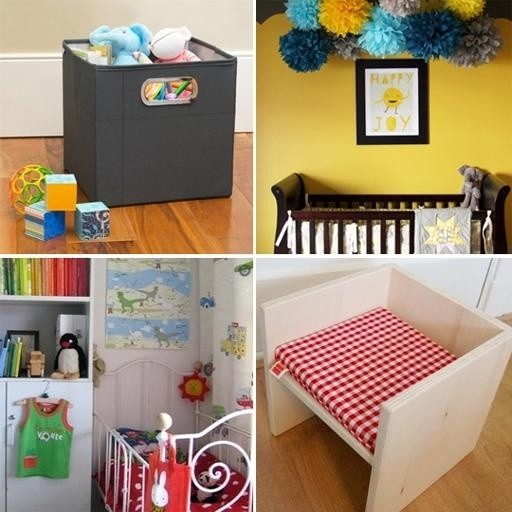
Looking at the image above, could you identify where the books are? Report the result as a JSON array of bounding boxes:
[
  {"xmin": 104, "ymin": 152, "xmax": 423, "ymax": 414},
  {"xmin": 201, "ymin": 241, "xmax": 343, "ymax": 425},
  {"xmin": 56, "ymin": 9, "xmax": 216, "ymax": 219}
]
[{"xmin": 1, "ymin": 257, "xmax": 90, "ymax": 297}]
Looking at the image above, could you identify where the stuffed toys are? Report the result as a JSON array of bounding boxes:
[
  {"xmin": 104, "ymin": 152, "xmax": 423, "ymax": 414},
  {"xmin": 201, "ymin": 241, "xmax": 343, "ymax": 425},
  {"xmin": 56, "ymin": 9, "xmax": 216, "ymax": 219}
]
[
  {"xmin": 72, "ymin": 19, "xmax": 206, "ymax": 105},
  {"xmin": 190, "ymin": 469, "xmax": 224, "ymax": 503},
  {"xmin": 49, "ymin": 332, "xmax": 88, "ymax": 379},
  {"xmin": 178, "ymin": 367, "xmax": 210, "ymax": 403},
  {"xmin": 457, "ymin": 164, "xmax": 486, "ymax": 215}
]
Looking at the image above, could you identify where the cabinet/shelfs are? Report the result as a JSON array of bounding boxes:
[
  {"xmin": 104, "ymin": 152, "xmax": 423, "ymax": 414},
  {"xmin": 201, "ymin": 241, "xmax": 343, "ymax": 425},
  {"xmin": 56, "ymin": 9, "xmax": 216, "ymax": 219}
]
[{"xmin": 0, "ymin": 254, "xmax": 94, "ymax": 512}]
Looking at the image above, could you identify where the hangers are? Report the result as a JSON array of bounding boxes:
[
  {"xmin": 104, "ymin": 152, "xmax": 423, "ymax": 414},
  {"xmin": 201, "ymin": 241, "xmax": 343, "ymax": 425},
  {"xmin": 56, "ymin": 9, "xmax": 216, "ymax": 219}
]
[{"xmin": 14, "ymin": 380, "xmax": 74, "ymax": 408}]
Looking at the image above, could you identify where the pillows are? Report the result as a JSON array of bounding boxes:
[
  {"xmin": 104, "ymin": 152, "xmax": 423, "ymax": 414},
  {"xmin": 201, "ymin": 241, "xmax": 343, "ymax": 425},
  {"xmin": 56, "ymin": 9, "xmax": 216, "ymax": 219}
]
[{"xmin": 116, "ymin": 427, "xmax": 180, "ymax": 464}]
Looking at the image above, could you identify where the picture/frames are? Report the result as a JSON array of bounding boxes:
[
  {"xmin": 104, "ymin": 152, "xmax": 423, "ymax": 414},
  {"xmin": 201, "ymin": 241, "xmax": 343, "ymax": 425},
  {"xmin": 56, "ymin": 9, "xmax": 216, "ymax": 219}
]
[
  {"xmin": 354, "ymin": 59, "xmax": 431, "ymax": 146},
  {"xmin": 6, "ymin": 330, "xmax": 40, "ymax": 373}
]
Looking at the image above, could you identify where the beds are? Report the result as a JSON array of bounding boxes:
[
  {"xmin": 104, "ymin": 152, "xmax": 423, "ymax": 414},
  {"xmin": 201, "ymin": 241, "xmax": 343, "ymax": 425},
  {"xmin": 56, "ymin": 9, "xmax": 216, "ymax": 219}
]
[
  {"xmin": 94, "ymin": 359, "xmax": 256, "ymax": 512},
  {"xmin": 271, "ymin": 172, "xmax": 511, "ymax": 254}
]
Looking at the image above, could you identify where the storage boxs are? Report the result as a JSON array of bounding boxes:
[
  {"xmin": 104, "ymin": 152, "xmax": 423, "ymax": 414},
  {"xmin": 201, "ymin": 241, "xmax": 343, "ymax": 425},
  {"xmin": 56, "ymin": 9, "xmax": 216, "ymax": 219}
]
[{"xmin": 63, "ymin": 37, "xmax": 237, "ymax": 209}]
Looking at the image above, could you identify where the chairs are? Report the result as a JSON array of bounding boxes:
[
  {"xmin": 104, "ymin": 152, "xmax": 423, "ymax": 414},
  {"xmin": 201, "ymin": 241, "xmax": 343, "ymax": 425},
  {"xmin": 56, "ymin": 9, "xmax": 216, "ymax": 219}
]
[{"xmin": 259, "ymin": 263, "xmax": 512, "ymax": 512}]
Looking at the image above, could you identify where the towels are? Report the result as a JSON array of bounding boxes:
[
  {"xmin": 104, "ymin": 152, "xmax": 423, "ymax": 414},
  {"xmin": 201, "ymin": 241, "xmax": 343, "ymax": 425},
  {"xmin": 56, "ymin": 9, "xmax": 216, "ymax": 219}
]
[{"xmin": 414, "ymin": 207, "xmax": 473, "ymax": 254}]
